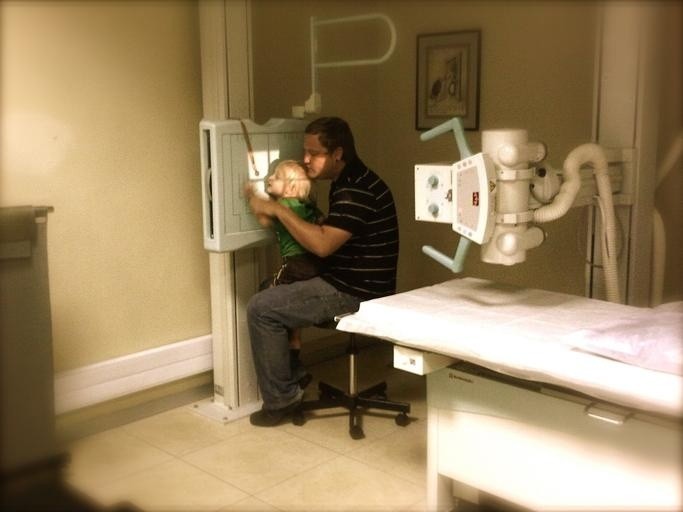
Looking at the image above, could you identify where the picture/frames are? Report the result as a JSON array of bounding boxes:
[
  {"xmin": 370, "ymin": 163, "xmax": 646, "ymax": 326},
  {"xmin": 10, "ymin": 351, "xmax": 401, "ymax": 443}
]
[{"xmin": 415, "ymin": 29, "xmax": 481, "ymax": 131}]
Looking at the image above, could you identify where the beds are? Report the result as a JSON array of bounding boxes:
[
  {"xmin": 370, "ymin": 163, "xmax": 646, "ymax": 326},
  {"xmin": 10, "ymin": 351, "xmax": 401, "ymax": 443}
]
[{"xmin": 334, "ymin": 278, "xmax": 683, "ymax": 511}]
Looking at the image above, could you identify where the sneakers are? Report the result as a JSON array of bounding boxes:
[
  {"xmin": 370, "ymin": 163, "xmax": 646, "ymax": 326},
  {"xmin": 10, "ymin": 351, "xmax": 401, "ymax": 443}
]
[{"xmin": 249, "ymin": 398, "xmax": 305, "ymax": 427}]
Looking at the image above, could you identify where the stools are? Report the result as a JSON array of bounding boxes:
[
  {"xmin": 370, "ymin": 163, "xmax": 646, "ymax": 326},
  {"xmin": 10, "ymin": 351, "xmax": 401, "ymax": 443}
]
[{"xmin": 290, "ymin": 315, "xmax": 411, "ymax": 439}]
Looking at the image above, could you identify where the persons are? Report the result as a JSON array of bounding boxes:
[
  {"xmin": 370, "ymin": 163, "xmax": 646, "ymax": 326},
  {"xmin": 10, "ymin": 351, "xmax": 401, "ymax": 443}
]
[
  {"xmin": 247, "ymin": 116, "xmax": 400, "ymax": 427},
  {"xmin": 244, "ymin": 159, "xmax": 332, "ymax": 363}
]
